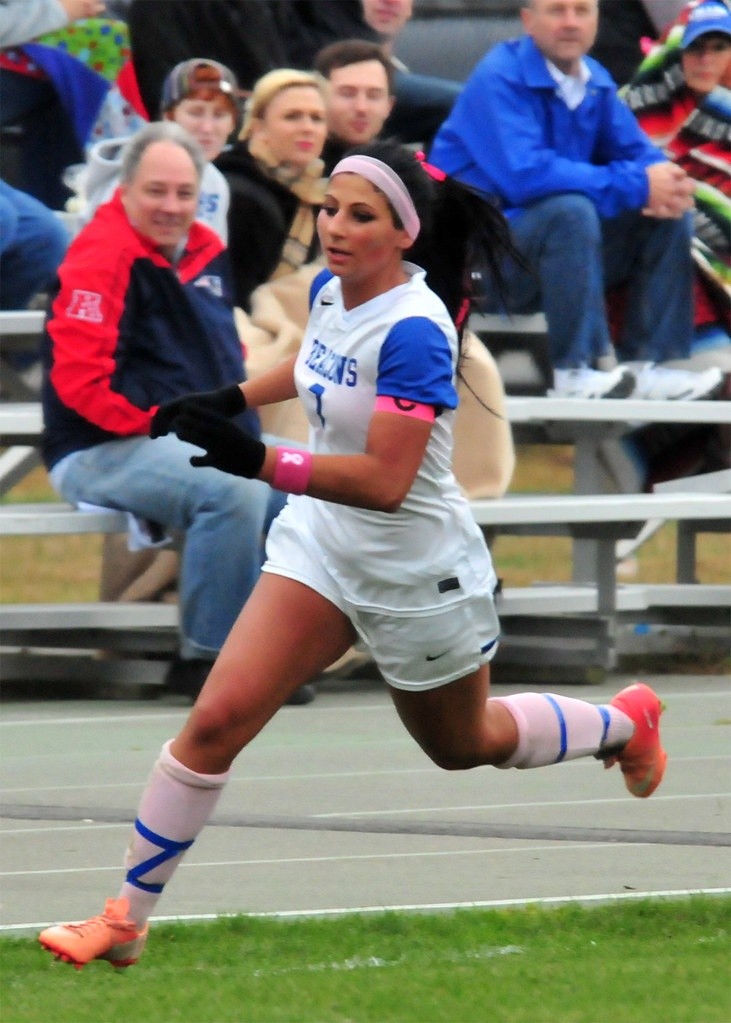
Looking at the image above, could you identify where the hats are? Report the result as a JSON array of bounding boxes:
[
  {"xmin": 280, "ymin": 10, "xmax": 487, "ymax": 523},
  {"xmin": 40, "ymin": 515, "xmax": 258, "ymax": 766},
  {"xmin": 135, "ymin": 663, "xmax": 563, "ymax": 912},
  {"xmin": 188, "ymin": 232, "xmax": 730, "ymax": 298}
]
[
  {"xmin": 678, "ymin": 0, "xmax": 731, "ymax": 52},
  {"xmin": 160, "ymin": 57, "xmax": 242, "ymax": 113}
]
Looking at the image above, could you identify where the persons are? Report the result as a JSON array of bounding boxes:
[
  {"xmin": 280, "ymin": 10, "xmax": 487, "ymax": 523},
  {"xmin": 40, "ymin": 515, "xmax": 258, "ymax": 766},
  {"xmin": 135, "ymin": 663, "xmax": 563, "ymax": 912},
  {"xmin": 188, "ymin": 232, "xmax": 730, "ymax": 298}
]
[
  {"xmin": 0, "ymin": 0, "xmax": 106, "ymax": 311},
  {"xmin": 82, "ymin": 0, "xmax": 414, "ymax": 309},
  {"xmin": 617, "ymin": 0, "xmax": 731, "ymax": 339},
  {"xmin": 428, "ymin": 0, "xmax": 723, "ymax": 401},
  {"xmin": 40, "ymin": 119, "xmax": 317, "ymax": 706},
  {"xmin": 40, "ymin": 143, "xmax": 668, "ymax": 974}
]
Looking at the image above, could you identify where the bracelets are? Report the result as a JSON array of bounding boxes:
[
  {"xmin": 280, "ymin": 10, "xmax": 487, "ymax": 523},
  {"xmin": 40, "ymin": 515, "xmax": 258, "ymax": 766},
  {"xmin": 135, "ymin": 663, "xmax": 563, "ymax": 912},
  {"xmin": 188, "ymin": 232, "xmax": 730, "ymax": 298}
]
[{"xmin": 269, "ymin": 445, "xmax": 313, "ymax": 496}]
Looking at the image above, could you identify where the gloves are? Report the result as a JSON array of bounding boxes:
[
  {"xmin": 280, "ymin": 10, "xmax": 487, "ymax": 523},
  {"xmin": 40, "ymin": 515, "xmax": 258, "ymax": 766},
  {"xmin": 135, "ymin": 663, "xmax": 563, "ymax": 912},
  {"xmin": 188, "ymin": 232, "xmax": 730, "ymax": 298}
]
[
  {"xmin": 149, "ymin": 403, "xmax": 266, "ymax": 479},
  {"xmin": 173, "ymin": 382, "xmax": 246, "ymax": 421}
]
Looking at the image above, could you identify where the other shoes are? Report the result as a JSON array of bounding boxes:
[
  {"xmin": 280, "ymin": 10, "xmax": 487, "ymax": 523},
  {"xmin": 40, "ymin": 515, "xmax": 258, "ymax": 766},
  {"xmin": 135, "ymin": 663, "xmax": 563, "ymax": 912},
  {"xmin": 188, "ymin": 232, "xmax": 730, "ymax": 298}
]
[
  {"xmin": 164, "ymin": 654, "xmax": 216, "ymax": 695},
  {"xmin": 279, "ymin": 684, "xmax": 317, "ymax": 707}
]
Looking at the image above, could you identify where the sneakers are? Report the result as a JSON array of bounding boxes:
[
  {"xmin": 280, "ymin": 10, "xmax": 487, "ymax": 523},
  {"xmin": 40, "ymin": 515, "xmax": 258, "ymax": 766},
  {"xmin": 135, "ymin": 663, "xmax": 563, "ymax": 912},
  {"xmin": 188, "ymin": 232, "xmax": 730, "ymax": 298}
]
[
  {"xmin": 39, "ymin": 898, "xmax": 149, "ymax": 967},
  {"xmin": 591, "ymin": 680, "xmax": 665, "ymax": 798},
  {"xmin": 546, "ymin": 357, "xmax": 637, "ymax": 401},
  {"xmin": 623, "ymin": 359, "xmax": 724, "ymax": 399}
]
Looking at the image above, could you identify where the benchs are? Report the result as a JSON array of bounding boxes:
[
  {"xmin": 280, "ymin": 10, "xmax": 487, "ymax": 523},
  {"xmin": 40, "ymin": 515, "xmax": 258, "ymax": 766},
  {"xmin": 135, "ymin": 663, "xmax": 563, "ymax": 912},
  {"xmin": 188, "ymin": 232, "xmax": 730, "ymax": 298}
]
[{"xmin": 0, "ymin": 311, "xmax": 731, "ymax": 700}]
